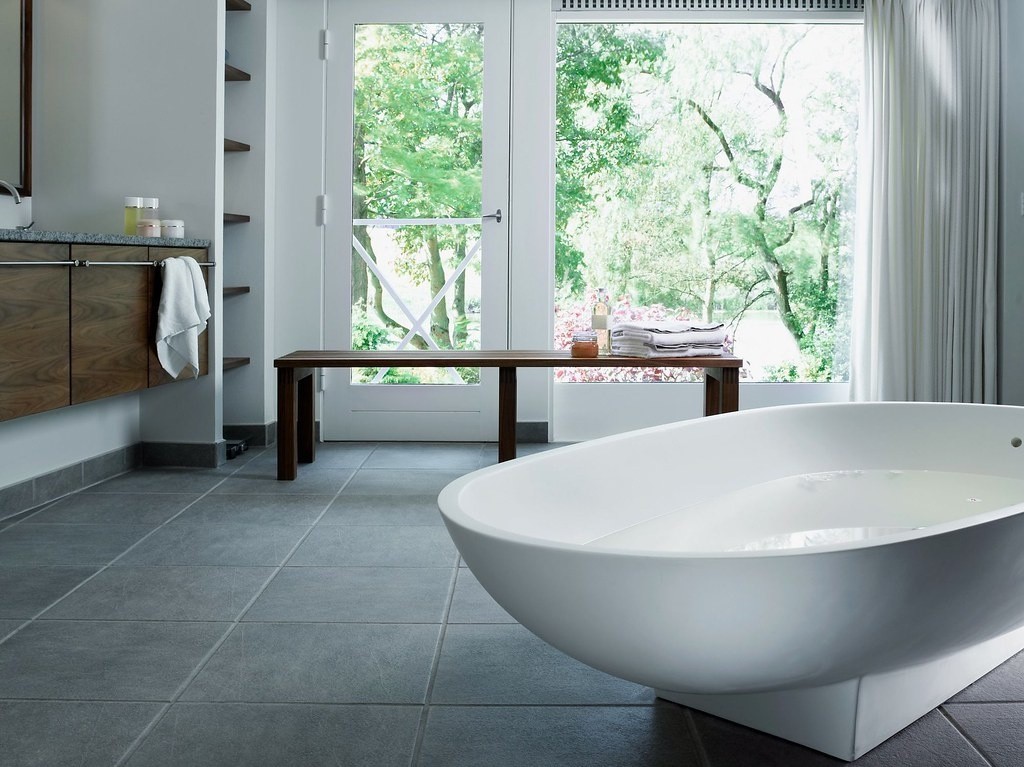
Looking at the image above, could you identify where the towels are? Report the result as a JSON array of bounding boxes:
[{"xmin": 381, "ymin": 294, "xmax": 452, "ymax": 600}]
[
  {"xmin": 154, "ymin": 254, "xmax": 212, "ymax": 381},
  {"xmin": 609, "ymin": 319, "xmax": 727, "ymax": 360}
]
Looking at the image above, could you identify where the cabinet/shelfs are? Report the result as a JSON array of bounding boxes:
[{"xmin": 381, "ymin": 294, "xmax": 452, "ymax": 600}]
[
  {"xmin": 222, "ymin": 0, "xmax": 255, "ymax": 462},
  {"xmin": 0, "ymin": 242, "xmax": 215, "ymax": 423}
]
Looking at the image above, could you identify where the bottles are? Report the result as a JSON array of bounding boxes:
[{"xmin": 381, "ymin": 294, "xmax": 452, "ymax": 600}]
[{"xmin": 592, "ymin": 289, "xmax": 611, "ymax": 355}]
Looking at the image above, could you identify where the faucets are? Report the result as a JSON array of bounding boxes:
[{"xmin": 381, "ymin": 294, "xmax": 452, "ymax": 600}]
[{"xmin": 0, "ymin": 178, "xmax": 22, "ymax": 205}]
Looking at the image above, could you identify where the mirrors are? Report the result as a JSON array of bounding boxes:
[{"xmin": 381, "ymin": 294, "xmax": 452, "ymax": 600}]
[{"xmin": 0, "ymin": 0, "xmax": 33, "ymax": 196}]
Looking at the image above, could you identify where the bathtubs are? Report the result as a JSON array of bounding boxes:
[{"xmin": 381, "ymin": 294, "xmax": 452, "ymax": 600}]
[{"xmin": 436, "ymin": 400, "xmax": 1024, "ymax": 763}]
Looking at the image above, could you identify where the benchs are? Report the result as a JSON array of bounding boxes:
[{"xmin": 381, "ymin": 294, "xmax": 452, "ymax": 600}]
[{"xmin": 272, "ymin": 350, "xmax": 743, "ymax": 480}]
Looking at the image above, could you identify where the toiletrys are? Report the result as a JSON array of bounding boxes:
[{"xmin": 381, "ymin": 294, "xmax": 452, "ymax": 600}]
[{"xmin": 123, "ymin": 196, "xmax": 185, "ymax": 239}]
